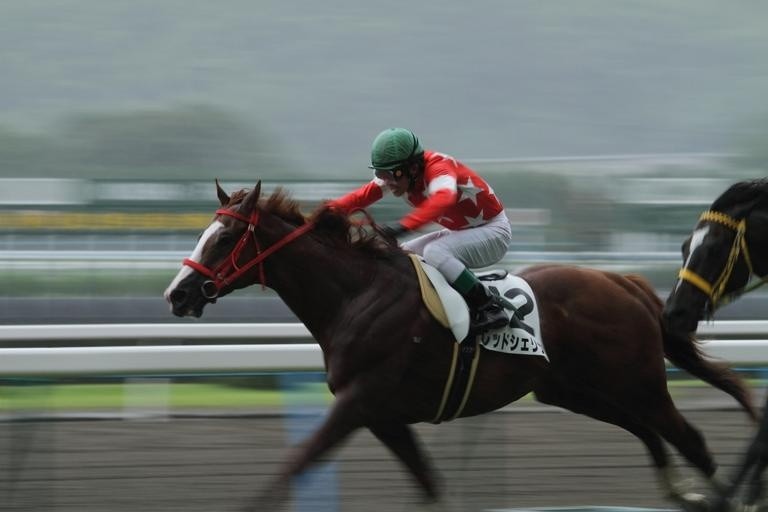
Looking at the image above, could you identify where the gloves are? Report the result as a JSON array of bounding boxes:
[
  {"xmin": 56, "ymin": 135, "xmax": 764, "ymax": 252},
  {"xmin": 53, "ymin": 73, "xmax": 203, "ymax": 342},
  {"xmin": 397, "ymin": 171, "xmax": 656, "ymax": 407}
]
[{"xmin": 376, "ymin": 222, "xmax": 406, "ymax": 240}]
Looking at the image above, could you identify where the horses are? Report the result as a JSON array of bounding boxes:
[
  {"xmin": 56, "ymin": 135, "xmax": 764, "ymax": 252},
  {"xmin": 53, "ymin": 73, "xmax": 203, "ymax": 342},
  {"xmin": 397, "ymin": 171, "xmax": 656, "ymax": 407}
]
[
  {"xmin": 656, "ymin": 174, "xmax": 768, "ymax": 511},
  {"xmin": 161, "ymin": 176, "xmax": 758, "ymax": 512}
]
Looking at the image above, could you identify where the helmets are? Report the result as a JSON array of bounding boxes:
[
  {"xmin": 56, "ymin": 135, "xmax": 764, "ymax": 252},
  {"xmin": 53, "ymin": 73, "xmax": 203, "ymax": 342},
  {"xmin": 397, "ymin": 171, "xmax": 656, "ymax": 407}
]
[{"xmin": 367, "ymin": 128, "xmax": 424, "ymax": 170}]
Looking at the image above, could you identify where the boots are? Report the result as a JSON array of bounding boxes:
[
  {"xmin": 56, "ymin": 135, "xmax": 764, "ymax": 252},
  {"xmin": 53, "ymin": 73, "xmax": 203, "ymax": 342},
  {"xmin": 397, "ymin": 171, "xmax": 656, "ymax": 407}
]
[{"xmin": 451, "ymin": 268, "xmax": 509, "ymax": 333}]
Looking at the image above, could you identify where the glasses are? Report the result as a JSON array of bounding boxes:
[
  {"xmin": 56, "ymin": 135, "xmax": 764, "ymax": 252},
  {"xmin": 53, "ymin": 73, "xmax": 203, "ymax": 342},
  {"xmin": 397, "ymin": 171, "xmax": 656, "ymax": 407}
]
[{"xmin": 375, "ymin": 169, "xmax": 404, "ymax": 179}]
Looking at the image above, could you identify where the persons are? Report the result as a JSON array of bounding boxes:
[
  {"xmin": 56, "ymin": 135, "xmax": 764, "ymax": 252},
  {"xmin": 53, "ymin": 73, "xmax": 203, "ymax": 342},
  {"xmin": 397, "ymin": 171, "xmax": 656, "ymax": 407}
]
[{"xmin": 322, "ymin": 128, "xmax": 511, "ymax": 336}]
